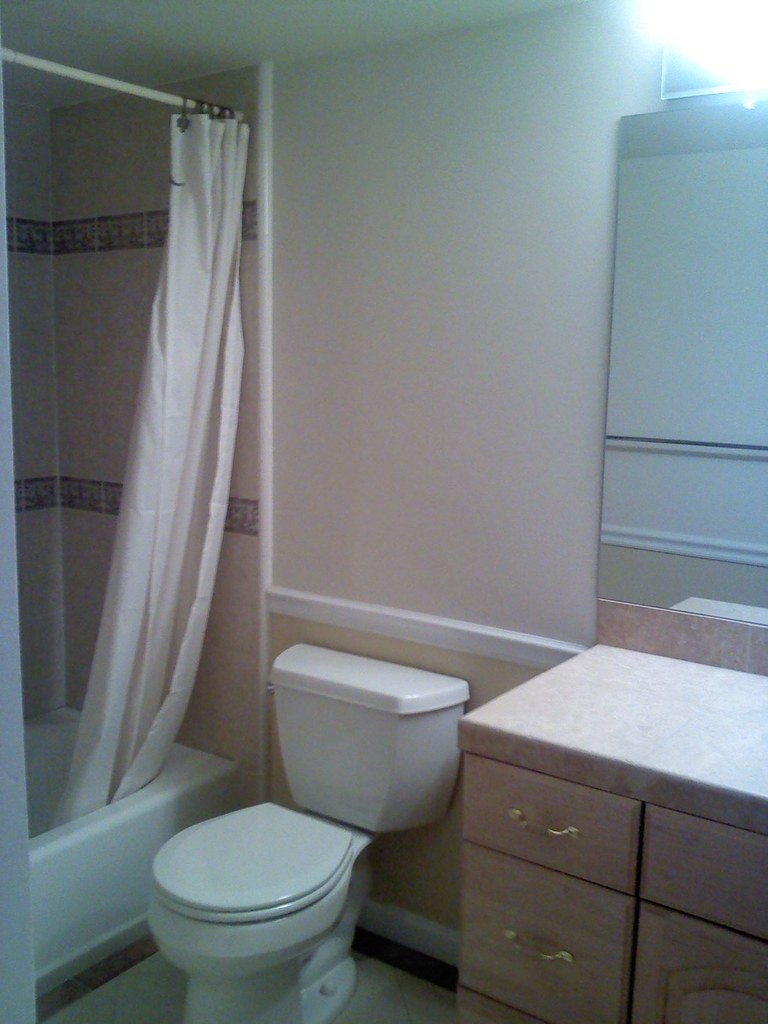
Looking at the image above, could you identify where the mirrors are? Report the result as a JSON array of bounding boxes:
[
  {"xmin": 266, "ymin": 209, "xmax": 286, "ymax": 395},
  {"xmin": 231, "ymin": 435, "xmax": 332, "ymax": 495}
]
[{"xmin": 593, "ymin": 98, "xmax": 768, "ymax": 675}]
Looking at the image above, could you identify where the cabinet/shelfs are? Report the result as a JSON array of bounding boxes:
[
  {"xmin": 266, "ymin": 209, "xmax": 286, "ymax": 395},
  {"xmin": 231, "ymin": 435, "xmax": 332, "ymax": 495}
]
[{"xmin": 455, "ymin": 645, "xmax": 768, "ymax": 1024}]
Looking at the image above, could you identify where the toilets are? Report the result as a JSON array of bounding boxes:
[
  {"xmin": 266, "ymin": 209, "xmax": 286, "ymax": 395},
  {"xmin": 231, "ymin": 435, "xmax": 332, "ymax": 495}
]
[{"xmin": 145, "ymin": 638, "xmax": 471, "ymax": 1022}]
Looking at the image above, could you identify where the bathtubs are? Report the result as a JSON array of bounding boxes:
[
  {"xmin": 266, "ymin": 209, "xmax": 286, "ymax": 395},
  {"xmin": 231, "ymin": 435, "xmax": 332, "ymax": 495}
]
[{"xmin": 23, "ymin": 704, "xmax": 239, "ymax": 1000}]
